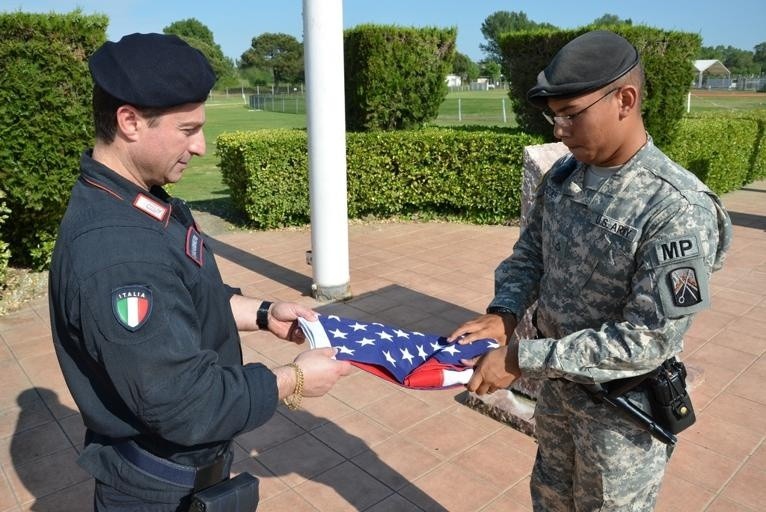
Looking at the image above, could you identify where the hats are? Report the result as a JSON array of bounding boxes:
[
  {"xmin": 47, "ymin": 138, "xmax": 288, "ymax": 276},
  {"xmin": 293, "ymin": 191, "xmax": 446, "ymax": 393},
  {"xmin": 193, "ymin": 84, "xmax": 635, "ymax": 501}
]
[
  {"xmin": 525, "ymin": 31, "xmax": 640, "ymax": 111},
  {"xmin": 88, "ymin": 32, "xmax": 216, "ymax": 107}
]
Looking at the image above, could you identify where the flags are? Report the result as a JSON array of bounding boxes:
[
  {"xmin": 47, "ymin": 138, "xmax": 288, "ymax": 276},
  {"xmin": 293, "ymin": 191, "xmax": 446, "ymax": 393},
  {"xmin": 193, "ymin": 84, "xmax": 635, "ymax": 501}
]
[{"xmin": 293, "ymin": 309, "xmax": 502, "ymax": 391}]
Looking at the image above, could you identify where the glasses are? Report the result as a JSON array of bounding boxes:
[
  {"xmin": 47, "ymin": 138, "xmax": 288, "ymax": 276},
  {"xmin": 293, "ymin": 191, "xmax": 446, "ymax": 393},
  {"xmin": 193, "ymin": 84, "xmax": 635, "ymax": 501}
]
[{"xmin": 540, "ymin": 88, "xmax": 619, "ymax": 126}]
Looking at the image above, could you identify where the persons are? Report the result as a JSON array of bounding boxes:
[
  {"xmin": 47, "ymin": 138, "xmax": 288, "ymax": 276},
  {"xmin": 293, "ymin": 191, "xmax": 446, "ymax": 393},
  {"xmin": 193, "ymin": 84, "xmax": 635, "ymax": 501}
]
[
  {"xmin": 46, "ymin": 31, "xmax": 351, "ymax": 512},
  {"xmin": 442, "ymin": 28, "xmax": 731, "ymax": 512}
]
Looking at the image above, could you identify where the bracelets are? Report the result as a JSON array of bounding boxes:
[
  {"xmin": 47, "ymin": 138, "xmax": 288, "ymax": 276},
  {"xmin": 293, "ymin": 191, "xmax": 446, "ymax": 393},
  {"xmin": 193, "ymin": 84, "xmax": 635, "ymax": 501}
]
[
  {"xmin": 279, "ymin": 363, "xmax": 305, "ymax": 413},
  {"xmin": 255, "ymin": 300, "xmax": 274, "ymax": 332}
]
